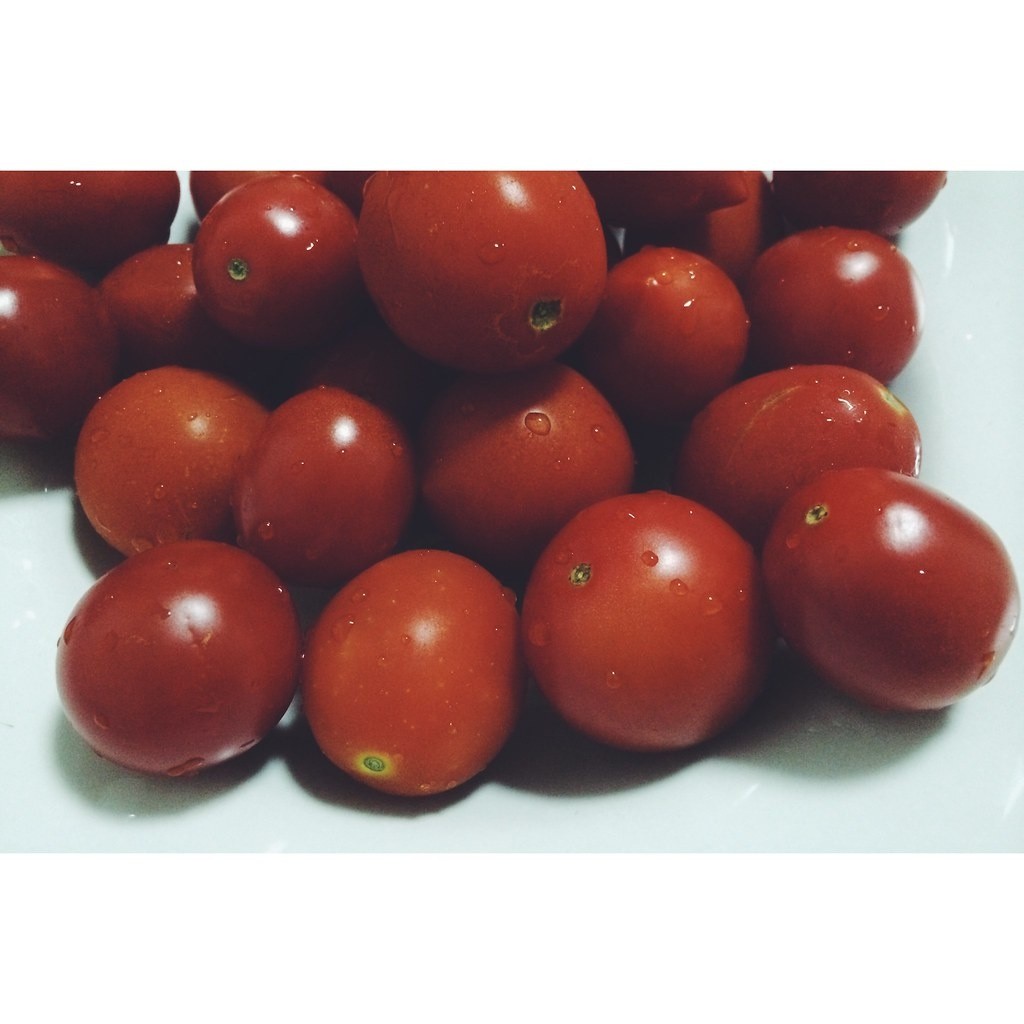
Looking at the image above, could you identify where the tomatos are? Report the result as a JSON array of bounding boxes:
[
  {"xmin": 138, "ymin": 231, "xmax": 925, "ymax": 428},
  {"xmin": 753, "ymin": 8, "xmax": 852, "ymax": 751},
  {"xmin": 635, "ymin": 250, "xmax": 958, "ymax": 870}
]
[{"xmin": 1, "ymin": 172, "xmax": 1021, "ymax": 798}]
